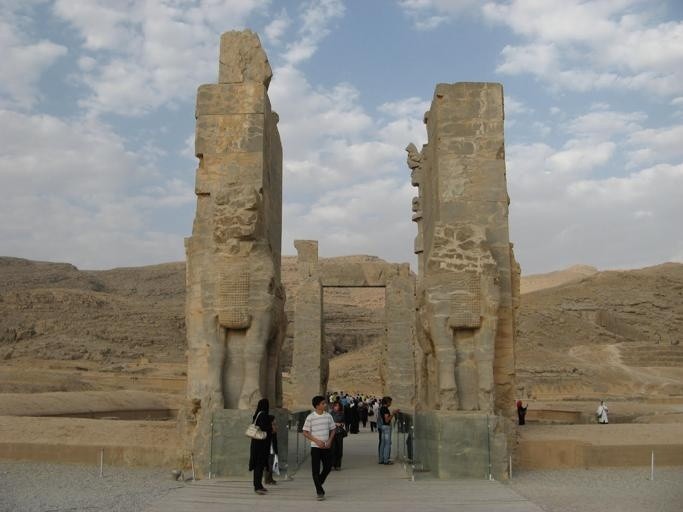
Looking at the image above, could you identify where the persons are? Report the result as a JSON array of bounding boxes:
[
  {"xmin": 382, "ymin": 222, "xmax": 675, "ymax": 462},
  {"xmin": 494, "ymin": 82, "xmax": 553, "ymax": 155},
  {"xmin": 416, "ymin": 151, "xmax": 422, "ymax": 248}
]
[
  {"xmin": 327, "ymin": 391, "xmax": 399, "ymax": 470},
  {"xmin": 596, "ymin": 400, "xmax": 609, "ymax": 424},
  {"xmin": 264, "ymin": 423, "xmax": 279, "ymax": 485},
  {"xmin": 517, "ymin": 401, "xmax": 528, "ymax": 425},
  {"xmin": 249, "ymin": 398, "xmax": 273, "ymax": 495},
  {"xmin": 302, "ymin": 396, "xmax": 337, "ymax": 500}
]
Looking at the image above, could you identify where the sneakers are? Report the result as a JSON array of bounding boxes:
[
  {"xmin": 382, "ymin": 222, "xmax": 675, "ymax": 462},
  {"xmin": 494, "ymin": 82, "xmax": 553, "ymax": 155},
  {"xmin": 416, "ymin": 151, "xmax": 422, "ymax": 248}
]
[
  {"xmin": 379, "ymin": 460, "xmax": 393, "ymax": 465},
  {"xmin": 255, "ymin": 479, "xmax": 277, "ymax": 495},
  {"xmin": 336, "ymin": 466, "xmax": 341, "ymax": 471},
  {"xmin": 317, "ymin": 488, "xmax": 325, "ymax": 501}
]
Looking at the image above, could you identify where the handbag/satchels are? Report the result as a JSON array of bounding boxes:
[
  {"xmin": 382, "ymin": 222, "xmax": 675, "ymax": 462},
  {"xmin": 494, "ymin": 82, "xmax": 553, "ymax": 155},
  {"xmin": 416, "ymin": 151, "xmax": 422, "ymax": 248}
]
[
  {"xmin": 273, "ymin": 454, "xmax": 280, "ymax": 475},
  {"xmin": 245, "ymin": 424, "xmax": 268, "ymax": 440},
  {"xmin": 335, "ymin": 426, "xmax": 347, "ymax": 438}
]
